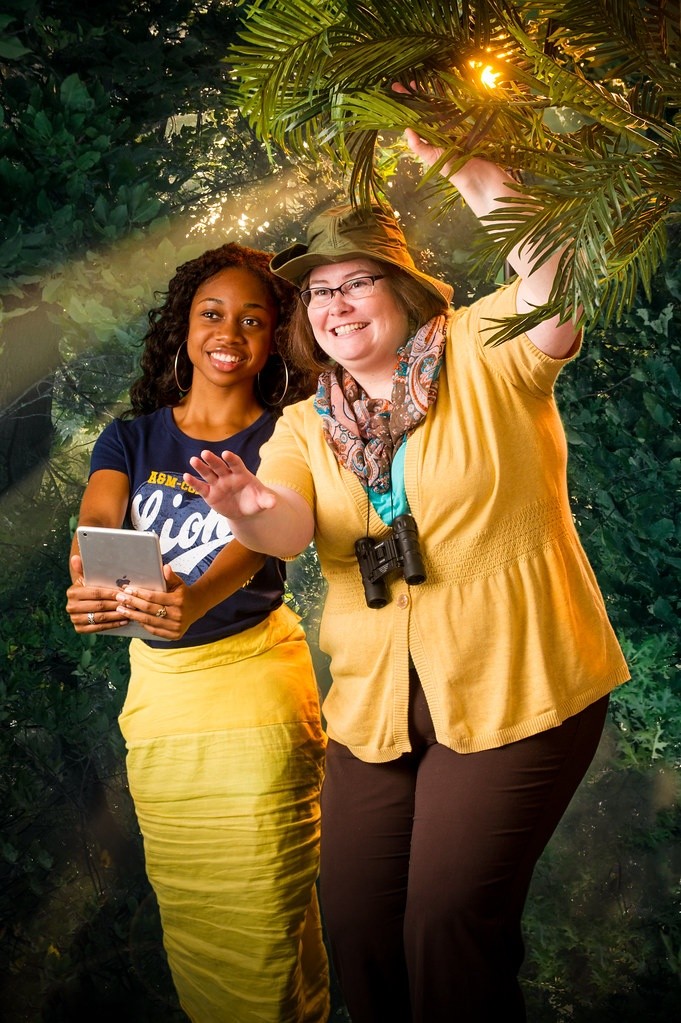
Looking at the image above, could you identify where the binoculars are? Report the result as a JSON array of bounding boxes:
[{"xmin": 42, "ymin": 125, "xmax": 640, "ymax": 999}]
[{"xmin": 355, "ymin": 514, "xmax": 426, "ymax": 610}]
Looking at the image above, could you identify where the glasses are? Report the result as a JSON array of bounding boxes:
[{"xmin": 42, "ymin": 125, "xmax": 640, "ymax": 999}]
[{"xmin": 299, "ymin": 273, "xmax": 387, "ymax": 309}]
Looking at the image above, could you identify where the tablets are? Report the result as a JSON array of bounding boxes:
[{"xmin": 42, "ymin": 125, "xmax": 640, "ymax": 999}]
[{"xmin": 76, "ymin": 526, "xmax": 173, "ymax": 642}]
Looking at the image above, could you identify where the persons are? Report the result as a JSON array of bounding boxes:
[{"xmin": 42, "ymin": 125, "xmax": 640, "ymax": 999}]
[
  {"xmin": 66, "ymin": 242, "xmax": 332, "ymax": 1023},
  {"xmin": 183, "ymin": 80, "xmax": 632, "ymax": 1023}
]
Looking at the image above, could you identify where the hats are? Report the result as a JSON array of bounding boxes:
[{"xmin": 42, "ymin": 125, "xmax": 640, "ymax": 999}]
[{"xmin": 267, "ymin": 200, "xmax": 454, "ymax": 310}]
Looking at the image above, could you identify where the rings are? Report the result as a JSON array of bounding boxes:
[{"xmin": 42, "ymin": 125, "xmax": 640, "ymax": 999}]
[
  {"xmin": 156, "ymin": 604, "xmax": 166, "ymax": 618},
  {"xmin": 88, "ymin": 613, "xmax": 95, "ymax": 626}
]
